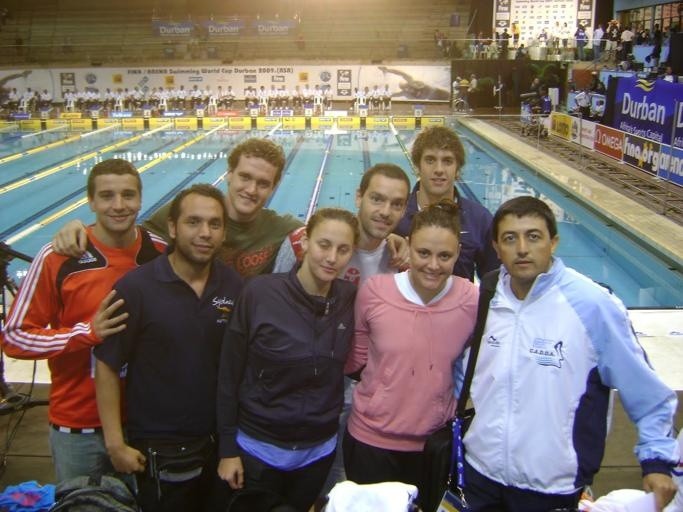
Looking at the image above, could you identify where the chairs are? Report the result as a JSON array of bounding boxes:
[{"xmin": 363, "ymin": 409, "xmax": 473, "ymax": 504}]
[
  {"xmin": 207, "ymin": 95, "xmax": 219, "ymax": 114},
  {"xmin": 313, "ymin": 95, "xmax": 325, "ymax": 115},
  {"xmin": 17, "ymin": 98, "xmax": 26, "ymax": 114},
  {"xmin": 64, "ymin": 98, "xmax": 75, "ymax": 112},
  {"xmin": 257, "ymin": 95, "xmax": 269, "ymax": 115},
  {"xmin": 157, "ymin": 96, "xmax": 168, "ymax": 111},
  {"xmin": 114, "ymin": 98, "xmax": 124, "ymax": 112},
  {"xmin": 356, "ymin": 95, "xmax": 367, "ymax": 114}
]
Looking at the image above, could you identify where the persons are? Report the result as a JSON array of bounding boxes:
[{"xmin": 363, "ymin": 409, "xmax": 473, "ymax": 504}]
[
  {"xmin": 51, "ymin": 137, "xmax": 411, "ymax": 280},
  {"xmin": 451, "ymin": 195, "xmax": 680, "ymax": 512},
  {"xmin": 529, "ymin": 22, "xmax": 674, "ymax": 123},
  {"xmin": 376, "ymin": 124, "xmax": 504, "ymax": 286},
  {"xmin": 92, "ymin": 182, "xmax": 247, "ymax": 512},
  {"xmin": 350, "ymin": 85, "xmax": 392, "ymax": 111},
  {"xmin": 433, "ymin": 23, "xmax": 525, "ymax": 111},
  {"xmin": 215, "ymin": 207, "xmax": 369, "ymax": 512},
  {"xmin": 8, "ymin": 87, "xmax": 52, "ymax": 112},
  {"xmin": 270, "ymin": 163, "xmax": 411, "ymax": 512},
  {"xmin": 63, "ymin": 84, "xmax": 334, "ymax": 118},
  {"xmin": 343, "ymin": 197, "xmax": 481, "ymax": 511},
  {"xmin": 1, "ymin": 157, "xmax": 170, "ymax": 511}
]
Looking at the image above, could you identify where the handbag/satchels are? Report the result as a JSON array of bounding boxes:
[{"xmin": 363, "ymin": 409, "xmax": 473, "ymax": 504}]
[
  {"xmin": 420, "ymin": 407, "xmax": 476, "ymax": 512},
  {"xmin": 149, "ymin": 439, "xmax": 206, "ymax": 501}
]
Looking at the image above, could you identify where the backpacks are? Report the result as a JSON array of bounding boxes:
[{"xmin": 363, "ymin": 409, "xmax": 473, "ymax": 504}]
[{"xmin": 48, "ymin": 473, "xmax": 141, "ymax": 512}]
